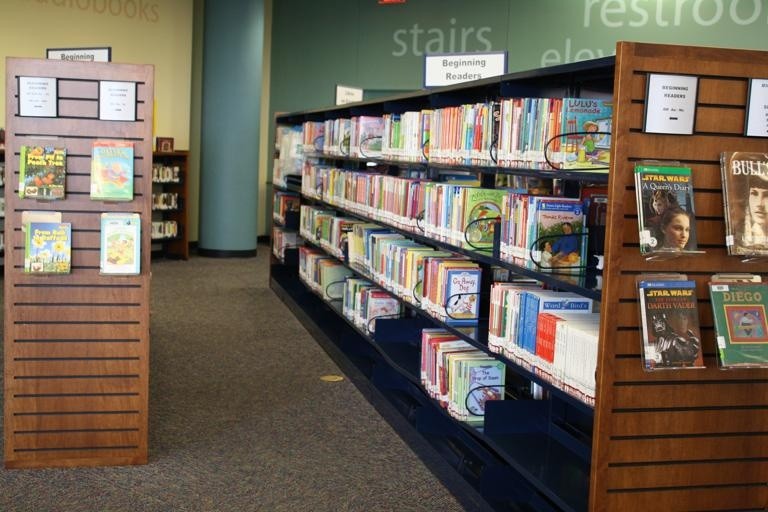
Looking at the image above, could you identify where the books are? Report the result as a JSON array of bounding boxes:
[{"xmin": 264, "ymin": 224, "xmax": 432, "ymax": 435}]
[
  {"xmin": 271, "ymin": 191, "xmax": 299, "ymax": 226},
  {"xmin": 272, "ymin": 124, "xmax": 301, "ymax": 187},
  {"xmin": 634, "ymin": 165, "xmax": 698, "ymax": 254},
  {"xmin": 488, "ymin": 280, "xmax": 601, "ymax": 407},
  {"xmin": 100, "ymin": 212, "xmax": 141, "ymax": 276},
  {"xmin": 719, "ymin": 151, "xmax": 768, "ymax": 257},
  {"xmin": 299, "ymin": 247, "xmax": 351, "ymax": 302},
  {"xmin": 299, "ymin": 203, "xmax": 510, "ymax": 323},
  {"xmin": 89, "ymin": 141, "xmax": 135, "ymax": 202},
  {"xmin": 419, "ymin": 326, "xmax": 509, "ymax": 424},
  {"xmin": 151, "ymin": 220, "xmax": 177, "ymax": 239},
  {"xmin": 19, "ymin": 144, "xmax": 67, "ymax": 200},
  {"xmin": 273, "ymin": 226, "xmax": 298, "ymax": 264},
  {"xmin": 152, "ymin": 192, "xmax": 178, "ymax": 210},
  {"xmin": 24, "ymin": 222, "xmax": 72, "ymax": 275},
  {"xmin": 708, "ymin": 273, "xmax": 768, "ymax": 374},
  {"xmin": 340, "ymin": 277, "xmax": 404, "ymax": 337},
  {"xmin": 637, "ymin": 279, "xmax": 706, "ymax": 371},
  {"xmin": 153, "ymin": 163, "xmax": 181, "ymax": 183}
]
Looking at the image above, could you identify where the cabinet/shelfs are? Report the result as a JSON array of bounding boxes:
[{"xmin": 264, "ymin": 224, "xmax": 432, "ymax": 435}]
[
  {"xmin": 268, "ymin": 53, "xmax": 619, "ymax": 511},
  {"xmin": 150, "ymin": 151, "xmax": 189, "ymax": 261},
  {"xmin": 3, "ymin": 56, "xmax": 154, "ymax": 470},
  {"xmin": 587, "ymin": 37, "xmax": 768, "ymax": 512}
]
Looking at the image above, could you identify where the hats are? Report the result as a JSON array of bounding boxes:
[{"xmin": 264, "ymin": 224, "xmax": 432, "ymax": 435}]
[{"xmin": 582, "ymin": 120, "xmax": 597, "ymax": 130}]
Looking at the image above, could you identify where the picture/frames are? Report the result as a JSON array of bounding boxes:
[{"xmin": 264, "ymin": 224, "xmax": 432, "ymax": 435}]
[
  {"xmin": 46, "ymin": 46, "xmax": 112, "ymax": 63},
  {"xmin": 423, "ymin": 50, "xmax": 508, "ymax": 90}
]
